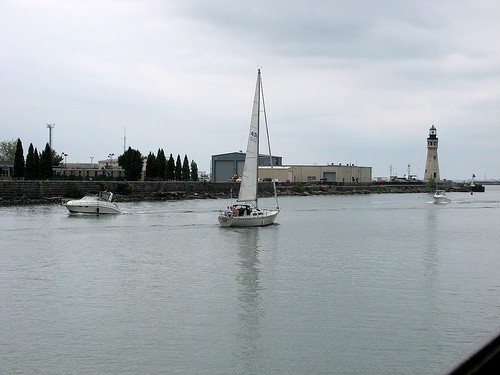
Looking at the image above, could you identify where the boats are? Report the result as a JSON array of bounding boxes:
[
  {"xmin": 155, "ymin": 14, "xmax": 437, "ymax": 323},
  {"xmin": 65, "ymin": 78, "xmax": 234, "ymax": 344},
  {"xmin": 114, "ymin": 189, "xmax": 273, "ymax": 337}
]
[
  {"xmin": 432, "ymin": 188, "xmax": 451, "ymax": 203},
  {"xmin": 63, "ymin": 190, "xmax": 123, "ymax": 215}
]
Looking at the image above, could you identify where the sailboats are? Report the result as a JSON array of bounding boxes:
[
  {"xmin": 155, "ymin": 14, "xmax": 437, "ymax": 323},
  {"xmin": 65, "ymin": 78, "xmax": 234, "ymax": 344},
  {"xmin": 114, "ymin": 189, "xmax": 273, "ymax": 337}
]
[{"xmin": 218, "ymin": 68, "xmax": 281, "ymax": 228}]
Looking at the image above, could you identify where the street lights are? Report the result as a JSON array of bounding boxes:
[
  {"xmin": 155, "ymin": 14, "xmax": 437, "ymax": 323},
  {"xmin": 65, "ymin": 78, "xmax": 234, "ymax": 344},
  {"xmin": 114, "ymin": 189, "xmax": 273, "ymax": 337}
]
[
  {"xmin": 109, "ymin": 153, "xmax": 115, "ymax": 164},
  {"xmin": 90, "ymin": 157, "xmax": 94, "ymax": 163},
  {"xmin": 45, "ymin": 122, "xmax": 56, "ymax": 147},
  {"xmin": 64, "ymin": 154, "xmax": 68, "ymax": 169}
]
